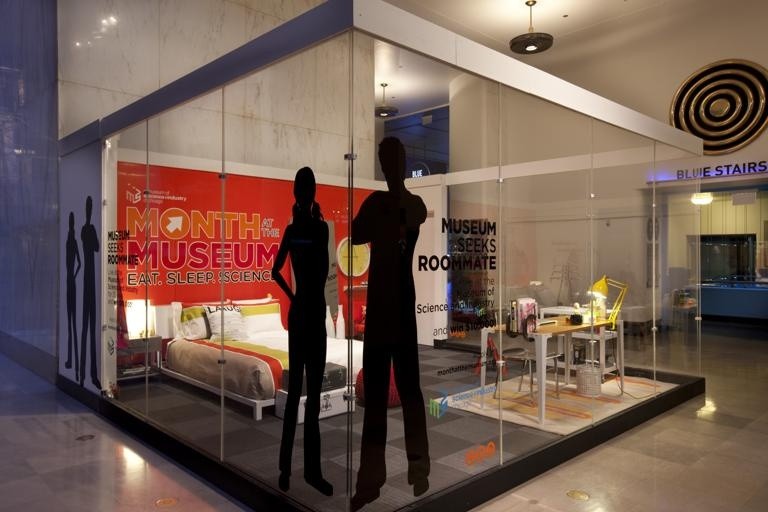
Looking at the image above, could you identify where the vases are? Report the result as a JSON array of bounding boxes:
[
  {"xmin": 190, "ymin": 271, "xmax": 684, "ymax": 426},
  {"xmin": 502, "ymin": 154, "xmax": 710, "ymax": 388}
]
[
  {"xmin": 324, "ymin": 304, "xmax": 335, "ymax": 338},
  {"xmin": 335, "ymin": 304, "xmax": 345, "ymax": 339}
]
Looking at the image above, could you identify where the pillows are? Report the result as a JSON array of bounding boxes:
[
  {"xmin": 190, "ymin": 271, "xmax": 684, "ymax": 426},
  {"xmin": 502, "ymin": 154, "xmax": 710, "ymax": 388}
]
[{"xmin": 167, "ymin": 298, "xmax": 283, "ymax": 342}]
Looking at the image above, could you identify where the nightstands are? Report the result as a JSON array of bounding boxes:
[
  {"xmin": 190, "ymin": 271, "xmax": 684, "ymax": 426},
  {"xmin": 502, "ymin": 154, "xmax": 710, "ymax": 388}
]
[{"xmin": 114, "ymin": 333, "xmax": 163, "ymax": 386}]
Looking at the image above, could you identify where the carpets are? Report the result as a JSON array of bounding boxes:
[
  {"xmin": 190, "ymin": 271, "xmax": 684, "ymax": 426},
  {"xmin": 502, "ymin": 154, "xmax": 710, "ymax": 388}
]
[{"xmin": 429, "ymin": 367, "xmax": 681, "ymax": 436}]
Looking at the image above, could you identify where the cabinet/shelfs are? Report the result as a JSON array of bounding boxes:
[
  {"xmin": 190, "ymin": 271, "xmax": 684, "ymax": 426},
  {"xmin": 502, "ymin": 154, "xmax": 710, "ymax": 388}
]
[{"xmin": 537, "ymin": 303, "xmax": 620, "ymax": 384}]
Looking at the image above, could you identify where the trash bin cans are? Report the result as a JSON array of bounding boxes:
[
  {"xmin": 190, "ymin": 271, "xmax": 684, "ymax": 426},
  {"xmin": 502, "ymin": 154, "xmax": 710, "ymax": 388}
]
[{"xmin": 575, "ymin": 364, "xmax": 601, "ymax": 398}]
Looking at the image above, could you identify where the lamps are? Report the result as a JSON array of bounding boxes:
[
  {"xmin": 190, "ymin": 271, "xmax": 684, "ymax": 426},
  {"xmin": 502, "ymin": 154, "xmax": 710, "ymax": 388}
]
[
  {"xmin": 373, "ymin": 83, "xmax": 399, "ymax": 119},
  {"xmin": 508, "ymin": 0, "xmax": 554, "ymax": 57},
  {"xmin": 586, "ymin": 271, "xmax": 632, "ymax": 332}
]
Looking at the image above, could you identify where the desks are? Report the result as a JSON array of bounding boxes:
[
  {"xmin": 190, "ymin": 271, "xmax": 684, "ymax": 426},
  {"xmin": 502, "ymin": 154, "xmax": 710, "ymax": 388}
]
[{"xmin": 475, "ymin": 311, "xmax": 626, "ymax": 428}]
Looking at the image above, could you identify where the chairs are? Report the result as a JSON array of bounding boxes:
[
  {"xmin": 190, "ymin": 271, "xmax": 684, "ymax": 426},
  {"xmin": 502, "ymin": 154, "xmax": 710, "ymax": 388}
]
[{"xmin": 486, "ymin": 329, "xmax": 563, "ymax": 402}]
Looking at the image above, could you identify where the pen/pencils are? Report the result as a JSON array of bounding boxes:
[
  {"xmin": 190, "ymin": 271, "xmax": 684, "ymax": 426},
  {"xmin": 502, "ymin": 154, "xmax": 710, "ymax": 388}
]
[{"xmin": 540, "ymin": 322, "xmax": 555, "ymax": 326}]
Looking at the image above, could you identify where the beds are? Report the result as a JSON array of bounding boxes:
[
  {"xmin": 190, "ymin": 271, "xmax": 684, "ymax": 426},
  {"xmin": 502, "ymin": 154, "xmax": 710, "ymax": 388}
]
[{"xmin": 152, "ymin": 297, "xmax": 365, "ymax": 424}]
